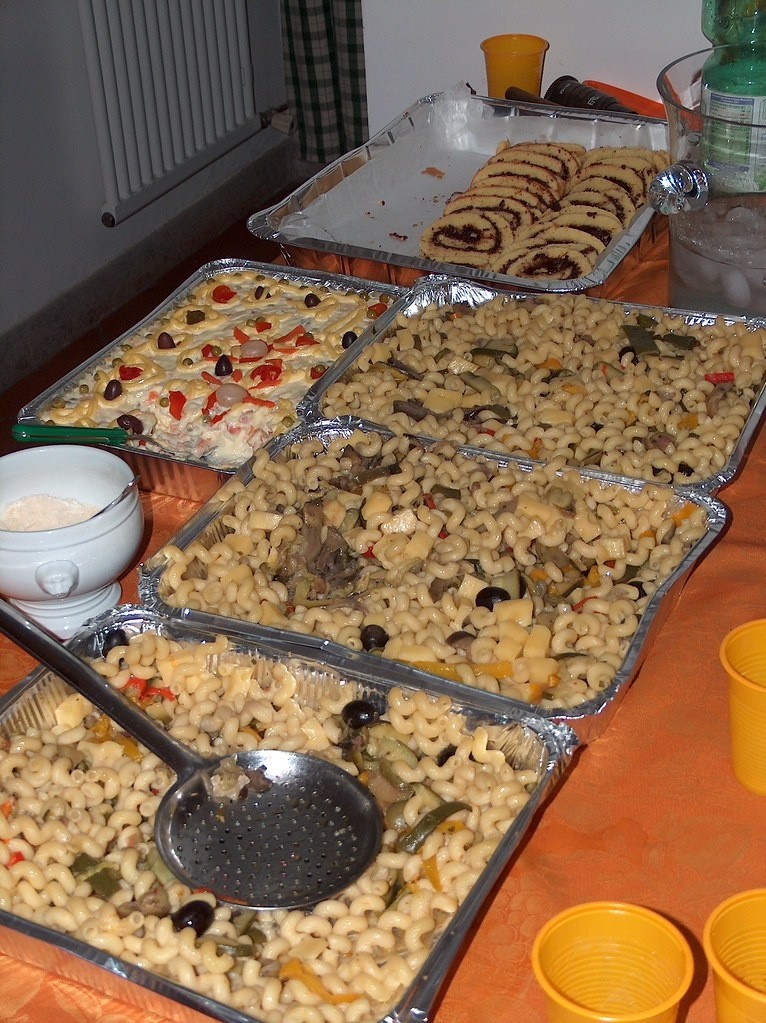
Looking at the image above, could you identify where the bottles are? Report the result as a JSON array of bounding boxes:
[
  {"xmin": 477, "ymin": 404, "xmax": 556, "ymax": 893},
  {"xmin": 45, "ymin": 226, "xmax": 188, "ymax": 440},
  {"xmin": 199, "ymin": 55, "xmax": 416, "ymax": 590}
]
[{"xmin": 700, "ymin": 0, "xmax": 766, "ymax": 196}]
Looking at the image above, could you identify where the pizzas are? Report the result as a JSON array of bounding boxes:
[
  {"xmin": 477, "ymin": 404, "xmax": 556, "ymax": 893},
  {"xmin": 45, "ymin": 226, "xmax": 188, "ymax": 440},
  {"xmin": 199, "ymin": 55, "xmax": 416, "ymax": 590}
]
[{"xmin": 34, "ymin": 270, "xmax": 396, "ymax": 467}]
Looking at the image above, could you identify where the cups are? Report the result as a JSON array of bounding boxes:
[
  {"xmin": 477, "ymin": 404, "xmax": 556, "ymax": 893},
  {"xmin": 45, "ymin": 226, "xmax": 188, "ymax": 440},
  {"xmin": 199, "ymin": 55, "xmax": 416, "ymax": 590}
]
[
  {"xmin": 719, "ymin": 617, "xmax": 766, "ymax": 796},
  {"xmin": 530, "ymin": 900, "xmax": 695, "ymax": 1023},
  {"xmin": 702, "ymin": 886, "xmax": 766, "ymax": 1022},
  {"xmin": 657, "ymin": 42, "xmax": 766, "ymax": 318},
  {"xmin": 479, "ymin": 34, "xmax": 550, "ymax": 103}
]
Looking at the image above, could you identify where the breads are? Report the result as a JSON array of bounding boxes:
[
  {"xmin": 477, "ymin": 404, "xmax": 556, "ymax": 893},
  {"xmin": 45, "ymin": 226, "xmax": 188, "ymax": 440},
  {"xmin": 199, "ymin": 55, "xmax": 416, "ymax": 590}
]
[{"xmin": 420, "ymin": 139, "xmax": 670, "ymax": 282}]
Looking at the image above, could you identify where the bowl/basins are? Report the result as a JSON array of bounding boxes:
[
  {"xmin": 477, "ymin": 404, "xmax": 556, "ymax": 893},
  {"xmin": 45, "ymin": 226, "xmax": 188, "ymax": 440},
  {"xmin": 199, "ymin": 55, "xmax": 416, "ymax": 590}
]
[{"xmin": 0, "ymin": 445, "xmax": 146, "ymax": 640}]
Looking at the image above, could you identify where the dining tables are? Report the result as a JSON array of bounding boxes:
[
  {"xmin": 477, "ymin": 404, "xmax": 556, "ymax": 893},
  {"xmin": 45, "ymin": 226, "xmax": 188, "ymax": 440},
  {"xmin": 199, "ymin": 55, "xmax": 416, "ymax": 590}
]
[{"xmin": 0, "ymin": 194, "xmax": 766, "ymax": 1021}]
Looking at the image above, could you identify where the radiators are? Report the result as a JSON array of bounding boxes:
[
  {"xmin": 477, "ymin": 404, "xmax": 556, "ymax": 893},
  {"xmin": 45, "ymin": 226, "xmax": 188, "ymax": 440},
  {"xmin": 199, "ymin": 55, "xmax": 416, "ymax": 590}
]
[{"xmin": 76, "ymin": 0, "xmax": 269, "ymax": 228}]
